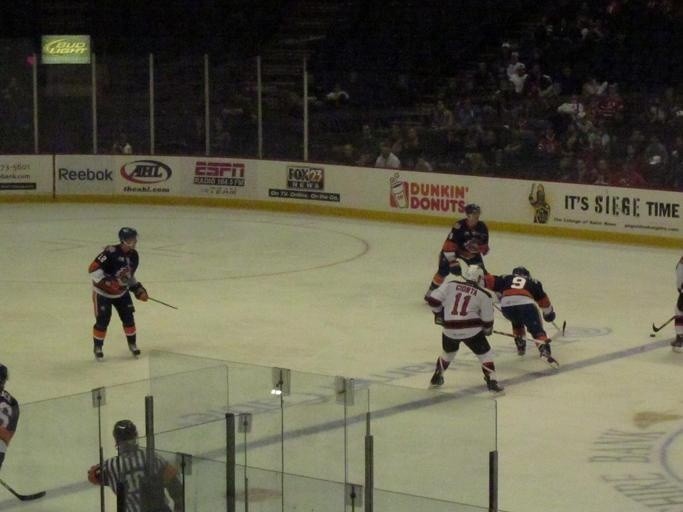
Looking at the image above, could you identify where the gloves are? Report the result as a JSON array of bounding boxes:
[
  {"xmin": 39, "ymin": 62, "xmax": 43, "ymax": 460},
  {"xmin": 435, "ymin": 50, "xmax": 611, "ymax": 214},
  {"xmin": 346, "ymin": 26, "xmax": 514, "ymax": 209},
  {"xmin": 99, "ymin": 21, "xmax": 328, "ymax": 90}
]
[
  {"xmin": 449, "ymin": 260, "xmax": 462, "ymax": 277},
  {"xmin": 102, "ymin": 278, "xmax": 120, "ymax": 293},
  {"xmin": 130, "ymin": 282, "xmax": 148, "ymax": 302},
  {"xmin": 543, "ymin": 307, "xmax": 556, "ymax": 323}
]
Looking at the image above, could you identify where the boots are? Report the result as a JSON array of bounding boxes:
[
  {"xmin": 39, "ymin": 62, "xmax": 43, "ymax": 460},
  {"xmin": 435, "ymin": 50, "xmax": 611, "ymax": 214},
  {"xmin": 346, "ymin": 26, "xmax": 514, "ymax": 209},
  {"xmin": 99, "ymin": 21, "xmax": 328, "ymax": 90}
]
[{"xmin": 670, "ymin": 336, "xmax": 682, "ymax": 346}]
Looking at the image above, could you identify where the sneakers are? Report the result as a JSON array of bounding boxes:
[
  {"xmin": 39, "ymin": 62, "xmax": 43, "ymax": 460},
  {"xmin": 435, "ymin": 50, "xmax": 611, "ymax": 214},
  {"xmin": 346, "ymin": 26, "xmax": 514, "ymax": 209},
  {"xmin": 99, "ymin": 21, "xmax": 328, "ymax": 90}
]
[
  {"xmin": 518, "ymin": 346, "xmax": 526, "ymax": 356},
  {"xmin": 540, "ymin": 353, "xmax": 559, "ymax": 368},
  {"xmin": 94, "ymin": 345, "xmax": 104, "ymax": 357},
  {"xmin": 129, "ymin": 343, "xmax": 141, "ymax": 355},
  {"xmin": 488, "ymin": 380, "xmax": 504, "ymax": 392},
  {"xmin": 431, "ymin": 374, "xmax": 445, "ymax": 386}
]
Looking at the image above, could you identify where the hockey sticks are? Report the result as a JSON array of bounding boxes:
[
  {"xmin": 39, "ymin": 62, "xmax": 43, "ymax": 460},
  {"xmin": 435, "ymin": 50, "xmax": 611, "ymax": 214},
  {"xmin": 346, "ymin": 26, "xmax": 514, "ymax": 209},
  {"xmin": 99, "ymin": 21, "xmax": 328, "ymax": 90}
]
[
  {"xmin": 0, "ymin": 478, "xmax": 45, "ymax": 501},
  {"xmin": 653, "ymin": 316, "xmax": 675, "ymax": 332},
  {"xmin": 550, "ymin": 320, "xmax": 566, "ymax": 336},
  {"xmin": 492, "ymin": 328, "xmax": 562, "ymax": 345}
]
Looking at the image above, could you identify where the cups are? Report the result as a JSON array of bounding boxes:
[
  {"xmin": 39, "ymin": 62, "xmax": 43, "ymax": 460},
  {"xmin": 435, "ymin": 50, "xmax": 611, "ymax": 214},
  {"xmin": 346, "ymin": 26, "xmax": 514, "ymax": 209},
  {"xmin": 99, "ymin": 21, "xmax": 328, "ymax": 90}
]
[{"xmin": 391, "ymin": 181, "xmax": 407, "ymax": 208}]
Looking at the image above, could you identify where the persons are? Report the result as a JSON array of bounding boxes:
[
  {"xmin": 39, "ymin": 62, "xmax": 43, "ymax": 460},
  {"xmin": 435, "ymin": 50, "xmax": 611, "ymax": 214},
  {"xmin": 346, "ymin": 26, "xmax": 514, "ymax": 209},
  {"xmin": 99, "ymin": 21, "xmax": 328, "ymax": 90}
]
[
  {"xmin": 426, "ymin": 263, "xmax": 504, "ymax": 391},
  {"xmin": 0, "ymin": 0, "xmax": 683, "ymax": 190},
  {"xmin": 0, "ymin": 362, "xmax": 20, "ymax": 474},
  {"xmin": 669, "ymin": 256, "xmax": 683, "ymax": 346},
  {"xmin": 484, "ymin": 266, "xmax": 560, "ymax": 369},
  {"xmin": 87, "ymin": 226, "xmax": 149, "ymax": 359},
  {"xmin": 423, "ymin": 203, "xmax": 490, "ymax": 301},
  {"xmin": 87, "ymin": 418, "xmax": 184, "ymax": 512}
]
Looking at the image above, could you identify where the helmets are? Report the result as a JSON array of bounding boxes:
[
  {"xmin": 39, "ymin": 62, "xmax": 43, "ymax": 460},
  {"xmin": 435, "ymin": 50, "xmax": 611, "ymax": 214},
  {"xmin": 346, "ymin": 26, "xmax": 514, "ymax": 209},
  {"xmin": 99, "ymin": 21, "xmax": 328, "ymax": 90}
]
[
  {"xmin": 1, "ymin": 364, "xmax": 6, "ymax": 384},
  {"xmin": 513, "ymin": 267, "xmax": 529, "ymax": 279},
  {"xmin": 114, "ymin": 420, "xmax": 137, "ymax": 443},
  {"xmin": 119, "ymin": 227, "xmax": 137, "ymax": 243},
  {"xmin": 465, "ymin": 203, "xmax": 481, "ymax": 215},
  {"xmin": 465, "ymin": 264, "xmax": 483, "ymax": 282}
]
